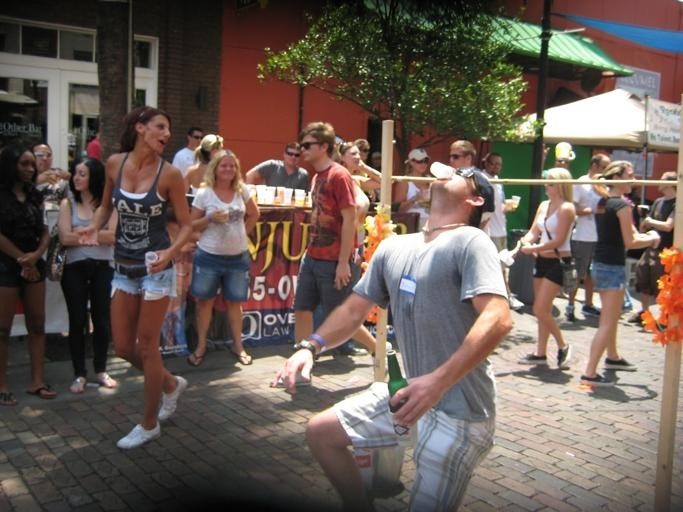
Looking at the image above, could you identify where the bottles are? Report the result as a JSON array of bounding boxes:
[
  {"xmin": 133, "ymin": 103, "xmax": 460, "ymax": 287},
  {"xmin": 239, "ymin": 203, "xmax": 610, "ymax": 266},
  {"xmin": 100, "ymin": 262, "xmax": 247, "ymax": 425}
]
[{"xmin": 385, "ymin": 349, "xmax": 420, "ymax": 449}]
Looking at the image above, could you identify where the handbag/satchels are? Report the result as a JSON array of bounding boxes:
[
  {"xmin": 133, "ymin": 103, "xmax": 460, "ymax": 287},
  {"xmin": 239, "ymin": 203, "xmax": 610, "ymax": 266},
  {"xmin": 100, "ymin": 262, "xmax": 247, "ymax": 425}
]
[
  {"xmin": 636, "ymin": 246, "xmax": 663, "ymax": 294},
  {"xmin": 48, "ymin": 225, "xmax": 67, "ymax": 281},
  {"xmin": 561, "ymin": 260, "xmax": 576, "ymax": 292}
]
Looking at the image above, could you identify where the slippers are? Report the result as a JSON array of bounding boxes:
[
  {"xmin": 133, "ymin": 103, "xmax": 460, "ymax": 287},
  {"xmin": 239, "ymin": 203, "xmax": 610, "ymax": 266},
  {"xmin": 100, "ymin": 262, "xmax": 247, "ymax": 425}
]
[
  {"xmin": 232, "ymin": 352, "xmax": 252, "ymax": 365},
  {"xmin": 0, "ymin": 371, "xmax": 117, "ymax": 405},
  {"xmin": 187, "ymin": 347, "xmax": 209, "ymax": 366}
]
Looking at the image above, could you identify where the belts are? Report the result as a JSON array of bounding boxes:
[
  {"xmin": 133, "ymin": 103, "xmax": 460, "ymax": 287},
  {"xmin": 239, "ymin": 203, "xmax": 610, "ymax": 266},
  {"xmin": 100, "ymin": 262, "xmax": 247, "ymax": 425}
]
[{"xmin": 115, "ymin": 261, "xmax": 172, "ymax": 277}]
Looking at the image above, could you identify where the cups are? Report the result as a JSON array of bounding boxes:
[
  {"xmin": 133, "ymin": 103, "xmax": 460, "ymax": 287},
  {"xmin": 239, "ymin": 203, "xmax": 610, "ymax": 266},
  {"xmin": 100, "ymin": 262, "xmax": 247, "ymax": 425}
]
[
  {"xmin": 145, "ymin": 251, "xmax": 158, "ymax": 271},
  {"xmin": 430, "ymin": 161, "xmax": 456, "ymax": 180},
  {"xmin": 244, "ymin": 183, "xmax": 313, "ymax": 205},
  {"xmin": 637, "ymin": 204, "xmax": 650, "ymax": 219},
  {"xmin": 504, "ymin": 195, "xmax": 521, "ymax": 210}
]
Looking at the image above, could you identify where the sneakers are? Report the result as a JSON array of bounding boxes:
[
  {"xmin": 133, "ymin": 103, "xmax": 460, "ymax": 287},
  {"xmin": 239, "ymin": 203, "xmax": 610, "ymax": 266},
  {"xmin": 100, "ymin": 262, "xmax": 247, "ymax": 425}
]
[
  {"xmin": 336, "ymin": 345, "xmax": 368, "ymax": 354},
  {"xmin": 629, "ymin": 311, "xmax": 644, "ymax": 322},
  {"xmin": 158, "ymin": 376, "xmax": 187, "ymax": 420},
  {"xmin": 117, "ymin": 420, "xmax": 161, "ymax": 449},
  {"xmin": 583, "ymin": 305, "xmax": 600, "ymax": 315},
  {"xmin": 581, "ymin": 373, "xmax": 614, "ymax": 386},
  {"xmin": 605, "ymin": 357, "xmax": 637, "ymax": 370},
  {"xmin": 566, "ymin": 305, "xmax": 574, "ymax": 320},
  {"xmin": 519, "ymin": 344, "xmax": 571, "ymax": 367}
]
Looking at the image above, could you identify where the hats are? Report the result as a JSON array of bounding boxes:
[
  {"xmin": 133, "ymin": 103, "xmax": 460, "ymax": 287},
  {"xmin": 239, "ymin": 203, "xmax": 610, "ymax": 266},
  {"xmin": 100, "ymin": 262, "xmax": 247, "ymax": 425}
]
[{"xmin": 408, "ymin": 148, "xmax": 431, "ymax": 161}]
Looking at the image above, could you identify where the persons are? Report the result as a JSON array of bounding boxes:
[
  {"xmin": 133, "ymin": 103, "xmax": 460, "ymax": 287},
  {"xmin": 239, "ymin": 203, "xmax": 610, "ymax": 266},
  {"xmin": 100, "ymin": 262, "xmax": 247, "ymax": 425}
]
[
  {"xmin": 0, "ymin": 142, "xmax": 56, "ymax": 407},
  {"xmin": 73, "ymin": 107, "xmax": 196, "ymax": 450},
  {"xmin": 31, "ymin": 120, "xmax": 263, "ymax": 408},
  {"xmin": 502, "ymin": 142, "xmax": 676, "ymax": 385},
  {"xmin": 240, "ymin": 122, "xmax": 389, "ymax": 391},
  {"xmin": 268, "ymin": 162, "xmax": 517, "ymax": 511},
  {"xmin": 394, "ymin": 142, "xmax": 526, "ymax": 313}
]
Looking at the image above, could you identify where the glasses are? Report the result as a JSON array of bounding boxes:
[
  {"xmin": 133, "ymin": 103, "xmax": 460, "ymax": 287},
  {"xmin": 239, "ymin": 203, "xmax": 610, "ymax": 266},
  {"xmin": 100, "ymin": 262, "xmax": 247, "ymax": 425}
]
[
  {"xmin": 300, "ymin": 142, "xmax": 320, "ymax": 149},
  {"xmin": 287, "ymin": 152, "xmax": 300, "ymax": 157},
  {"xmin": 35, "ymin": 151, "xmax": 52, "ymax": 158},
  {"xmin": 457, "ymin": 167, "xmax": 479, "ymax": 189},
  {"xmin": 194, "ymin": 136, "xmax": 202, "ymax": 139},
  {"xmin": 450, "ymin": 155, "xmax": 463, "ymax": 159}
]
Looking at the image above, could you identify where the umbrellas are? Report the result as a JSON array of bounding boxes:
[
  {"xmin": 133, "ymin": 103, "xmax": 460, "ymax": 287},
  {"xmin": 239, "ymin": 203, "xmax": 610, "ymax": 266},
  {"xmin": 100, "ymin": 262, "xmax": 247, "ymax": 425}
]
[{"xmin": 502, "ymin": 80, "xmax": 682, "ymax": 219}]
